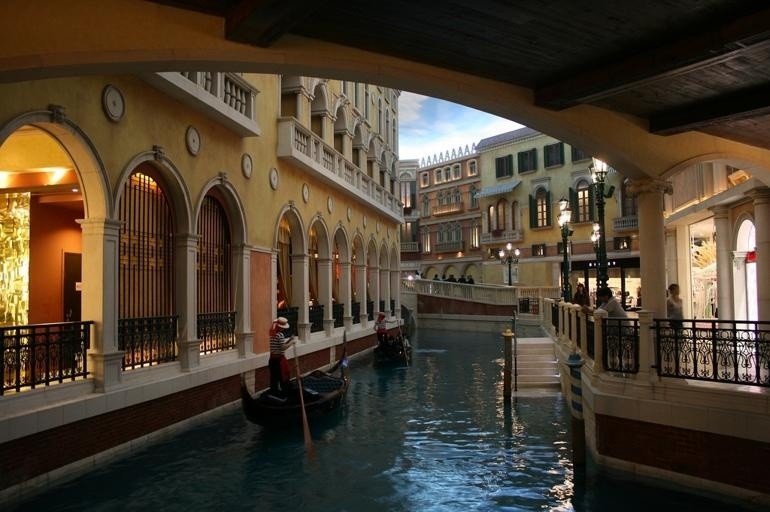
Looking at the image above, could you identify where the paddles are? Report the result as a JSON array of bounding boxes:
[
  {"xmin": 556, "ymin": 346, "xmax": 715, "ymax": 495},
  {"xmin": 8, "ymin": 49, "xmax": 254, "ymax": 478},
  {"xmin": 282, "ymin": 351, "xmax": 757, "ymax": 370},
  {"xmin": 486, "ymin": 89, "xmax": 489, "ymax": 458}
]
[
  {"xmin": 291, "ymin": 334, "xmax": 312, "ymax": 446},
  {"xmin": 397, "ymin": 320, "xmax": 409, "ymax": 366}
]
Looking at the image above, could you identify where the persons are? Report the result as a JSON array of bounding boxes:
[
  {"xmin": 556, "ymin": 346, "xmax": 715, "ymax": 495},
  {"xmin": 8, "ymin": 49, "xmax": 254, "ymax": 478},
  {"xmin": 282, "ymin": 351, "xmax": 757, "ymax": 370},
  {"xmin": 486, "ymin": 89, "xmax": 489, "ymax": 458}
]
[
  {"xmin": 414, "ymin": 270, "xmax": 474, "ymax": 284},
  {"xmin": 574, "ymin": 284, "xmax": 589, "ymax": 304},
  {"xmin": 269, "ymin": 316, "xmax": 297, "ymax": 391},
  {"xmin": 373, "ymin": 312, "xmax": 400, "ymax": 346},
  {"xmin": 593, "ymin": 287, "xmax": 632, "ymax": 326},
  {"xmin": 666, "ymin": 284, "xmax": 683, "ymax": 327}
]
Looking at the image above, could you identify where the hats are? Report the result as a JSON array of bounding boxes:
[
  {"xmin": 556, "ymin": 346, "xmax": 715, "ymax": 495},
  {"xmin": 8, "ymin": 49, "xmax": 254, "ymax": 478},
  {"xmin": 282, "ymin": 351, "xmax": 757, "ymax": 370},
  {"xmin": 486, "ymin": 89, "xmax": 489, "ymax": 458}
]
[{"xmin": 272, "ymin": 317, "xmax": 290, "ymax": 329}]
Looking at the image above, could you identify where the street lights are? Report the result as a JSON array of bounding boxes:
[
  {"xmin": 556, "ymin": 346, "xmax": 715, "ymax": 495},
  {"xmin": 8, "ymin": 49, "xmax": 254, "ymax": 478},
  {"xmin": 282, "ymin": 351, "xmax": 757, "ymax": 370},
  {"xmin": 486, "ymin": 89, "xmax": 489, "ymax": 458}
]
[
  {"xmin": 556, "ymin": 196, "xmax": 572, "ymax": 301},
  {"xmin": 498, "ymin": 243, "xmax": 521, "ymax": 284},
  {"xmin": 589, "ymin": 156, "xmax": 608, "ymax": 297}
]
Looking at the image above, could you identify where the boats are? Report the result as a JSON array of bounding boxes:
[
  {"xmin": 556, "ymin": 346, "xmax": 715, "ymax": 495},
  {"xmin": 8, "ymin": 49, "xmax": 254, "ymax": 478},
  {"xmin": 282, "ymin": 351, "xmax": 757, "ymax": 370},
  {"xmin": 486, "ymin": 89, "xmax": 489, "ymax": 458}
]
[
  {"xmin": 374, "ymin": 337, "xmax": 402, "ymax": 364},
  {"xmin": 240, "ymin": 368, "xmax": 349, "ymax": 427}
]
